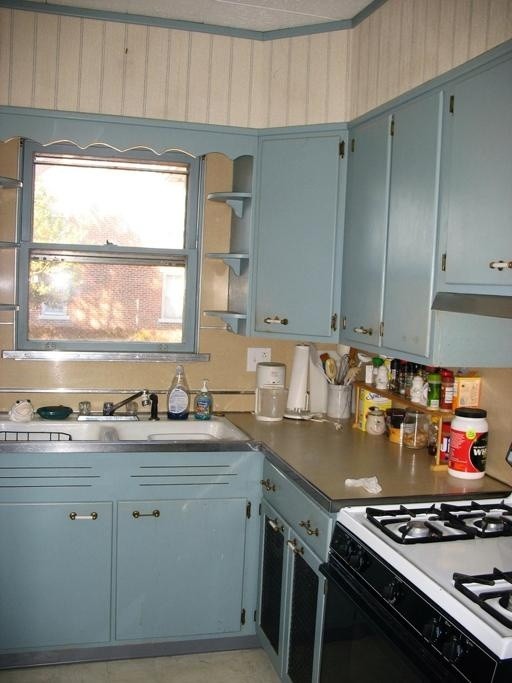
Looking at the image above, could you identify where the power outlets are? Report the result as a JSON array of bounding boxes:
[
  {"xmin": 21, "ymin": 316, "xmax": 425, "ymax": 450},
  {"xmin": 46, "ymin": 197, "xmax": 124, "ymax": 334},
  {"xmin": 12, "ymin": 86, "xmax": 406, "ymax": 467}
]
[{"xmin": 246, "ymin": 347, "xmax": 272, "ymax": 371}]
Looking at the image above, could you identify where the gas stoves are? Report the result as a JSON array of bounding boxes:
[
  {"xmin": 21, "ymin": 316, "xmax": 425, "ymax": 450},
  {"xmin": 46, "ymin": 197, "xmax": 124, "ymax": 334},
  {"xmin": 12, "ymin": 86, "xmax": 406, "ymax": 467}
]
[{"xmin": 321, "ymin": 493, "xmax": 509, "ymax": 682}]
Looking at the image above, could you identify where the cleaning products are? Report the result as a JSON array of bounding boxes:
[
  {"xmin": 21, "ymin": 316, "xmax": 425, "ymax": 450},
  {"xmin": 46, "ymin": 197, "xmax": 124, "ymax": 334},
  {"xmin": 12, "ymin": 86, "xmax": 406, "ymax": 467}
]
[
  {"xmin": 166, "ymin": 365, "xmax": 190, "ymax": 421},
  {"xmin": 193, "ymin": 379, "xmax": 213, "ymax": 421}
]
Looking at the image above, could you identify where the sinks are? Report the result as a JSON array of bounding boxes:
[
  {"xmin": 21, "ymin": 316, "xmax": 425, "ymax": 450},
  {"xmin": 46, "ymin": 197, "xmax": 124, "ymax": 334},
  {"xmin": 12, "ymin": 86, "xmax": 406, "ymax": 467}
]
[
  {"xmin": 112, "ymin": 418, "xmax": 242, "ymax": 446},
  {"xmin": 0, "ymin": 419, "xmax": 107, "ymax": 446}
]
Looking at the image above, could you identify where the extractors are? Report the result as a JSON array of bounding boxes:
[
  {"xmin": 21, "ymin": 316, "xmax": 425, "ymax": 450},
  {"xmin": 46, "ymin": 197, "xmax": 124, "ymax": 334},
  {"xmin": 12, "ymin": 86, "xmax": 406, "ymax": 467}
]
[{"xmin": 428, "ymin": 291, "xmax": 512, "ymax": 319}]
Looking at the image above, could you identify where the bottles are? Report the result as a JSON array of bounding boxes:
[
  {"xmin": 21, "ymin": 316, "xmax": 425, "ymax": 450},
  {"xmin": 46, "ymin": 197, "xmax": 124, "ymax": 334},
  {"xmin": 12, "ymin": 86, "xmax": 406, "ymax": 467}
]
[
  {"xmin": 427, "ymin": 374, "xmax": 441, "ymax": 408},
  {"xmin": 410, "ymin": 376, "xmax": 423, "ymax": 402},
  {"xmin": 372, "ymin": 358, "xmax": 383, "ymax": 386},
  {"xmin": 440, "ymin": 371, "xmax": 453, "ymax": 408},
  {"xmin": 428, "ymin": 416, "xmax": 439, "ymax": 455},
  {"xmin": 403, "ymin": 408, "xmax": 428, "ymax": 449},
  {"xmin": 375, "ymin": 366, "xmax": 388, "ymax": 389},
  {"xmin": 365, "ymin": 407, "xmax": 385, "ymax": 435},
  {"xmin": 448, "ymin": 407, "xmax": 489, "ymax": 479}
]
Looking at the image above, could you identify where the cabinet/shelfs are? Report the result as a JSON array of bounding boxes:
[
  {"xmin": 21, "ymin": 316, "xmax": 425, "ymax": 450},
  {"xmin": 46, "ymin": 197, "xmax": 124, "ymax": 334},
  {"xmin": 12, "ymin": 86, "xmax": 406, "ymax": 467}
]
[
  {"xmin": 0, "ymin": 176, "xmax": 24, "ymax": 311},
  {"xmin": 250, "ymin": 121, "xmax": 348, "ymax": 342},
  {"xmin": 203, "ymin": 191, "xmax": 251, "ymax": 337},
  {"xmin": 256, "ymin": 442, "xmax": 332, "ymax": 682},
  {"xmin": 434, "ymin": 40, "xmax": 511, "ymax": 296},
  {"xmin": 0, "ymin": 451, "xmax": 264, "ymax": 653},
  {"xmin": 339, "ymin": 37, "xmax": 512, "ymax": 367}
]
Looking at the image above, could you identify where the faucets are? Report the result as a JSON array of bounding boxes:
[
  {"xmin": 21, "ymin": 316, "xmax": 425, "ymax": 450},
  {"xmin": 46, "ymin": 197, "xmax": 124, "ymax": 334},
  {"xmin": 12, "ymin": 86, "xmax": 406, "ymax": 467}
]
[{"xmin": 102, "ymin": 388, "xmax": 151, "ymax": 416}]
